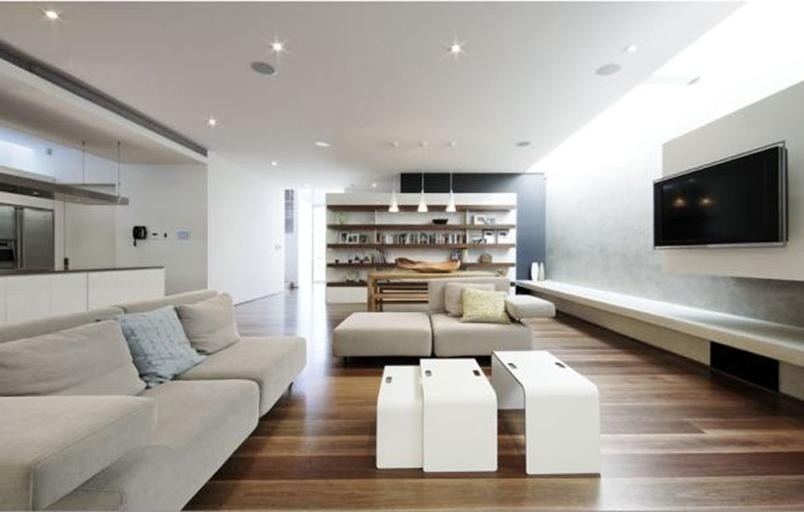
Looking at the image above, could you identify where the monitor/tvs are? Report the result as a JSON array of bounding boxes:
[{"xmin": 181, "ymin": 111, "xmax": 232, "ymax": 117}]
[{"xmin": 651, "ymin": 141, "xmax": 789, "ymax": 248}]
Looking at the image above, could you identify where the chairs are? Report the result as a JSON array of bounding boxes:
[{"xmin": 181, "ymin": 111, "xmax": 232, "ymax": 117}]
[{"xmin": 432, "ymin": 276, "xmax": 557, "ymax": 366}]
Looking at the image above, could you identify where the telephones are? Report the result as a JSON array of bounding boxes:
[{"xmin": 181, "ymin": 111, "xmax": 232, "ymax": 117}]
[{"xmin": 133, "ymin": 226, "xmax": 147, "ymax": 239}]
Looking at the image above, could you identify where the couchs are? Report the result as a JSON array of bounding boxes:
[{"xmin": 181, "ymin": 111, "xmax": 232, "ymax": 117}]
[{"xmin": 0, "ymin": 288, "xmax": 309, "ymax": 512}]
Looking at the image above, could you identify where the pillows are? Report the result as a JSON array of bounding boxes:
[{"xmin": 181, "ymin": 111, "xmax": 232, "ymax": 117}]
[
  {"xmin": 1, "ymin": 292, "xmax": 239, "ymax": 409},
  {"xmin": 443, "ymin": 281, "xmax": 512, "ymax": 325}
]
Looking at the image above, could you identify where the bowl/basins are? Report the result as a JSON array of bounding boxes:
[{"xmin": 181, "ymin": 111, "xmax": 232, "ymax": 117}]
[{"xmin": 433, "ymin": 219, "xmax": 448, "ymax": 225}]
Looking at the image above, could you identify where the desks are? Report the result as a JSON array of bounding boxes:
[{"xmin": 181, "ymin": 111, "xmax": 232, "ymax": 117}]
[{"xmin": 363, "ymin": 269, "xmax": 432, "ymax": 312}]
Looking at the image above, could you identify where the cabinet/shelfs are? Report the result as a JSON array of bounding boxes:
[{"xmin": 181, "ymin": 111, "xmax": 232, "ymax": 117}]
[{"xmin": 326, "ymin": 202, "xmax": 516, "ymax": 286}]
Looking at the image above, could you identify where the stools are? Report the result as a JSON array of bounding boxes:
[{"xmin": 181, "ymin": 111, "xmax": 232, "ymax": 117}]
[{"xmin": 331, "ymin": 310, "xmax": 432, "ymax": 366}]
